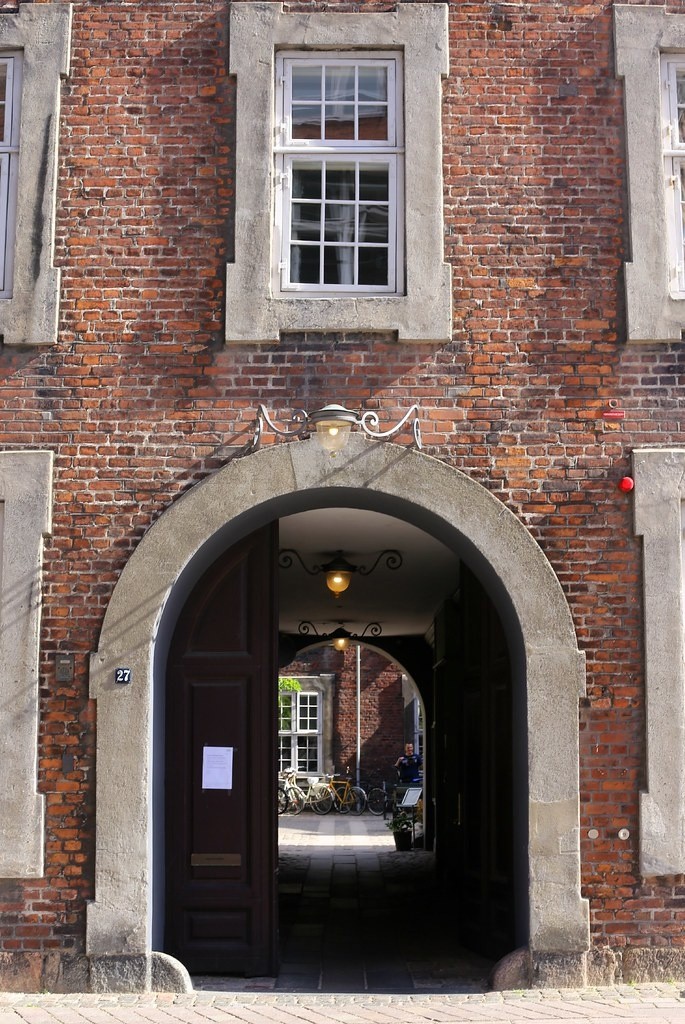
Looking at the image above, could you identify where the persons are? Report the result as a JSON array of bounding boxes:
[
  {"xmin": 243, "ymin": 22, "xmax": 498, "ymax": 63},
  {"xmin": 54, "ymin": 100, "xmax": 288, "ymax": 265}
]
[{"xmin": 393, "ymin": 741, "xmax": 422, "ymax": 785}]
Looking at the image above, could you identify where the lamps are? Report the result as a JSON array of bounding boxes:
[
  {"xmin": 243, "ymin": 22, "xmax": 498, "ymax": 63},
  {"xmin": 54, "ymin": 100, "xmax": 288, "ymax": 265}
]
[
  {"xmin": 279, "ymin": 544, "xmax": 403, "ymax": 598},
  {"xmin": 299, "ymin": 620, "xmax": 382, "ymax": 650}
]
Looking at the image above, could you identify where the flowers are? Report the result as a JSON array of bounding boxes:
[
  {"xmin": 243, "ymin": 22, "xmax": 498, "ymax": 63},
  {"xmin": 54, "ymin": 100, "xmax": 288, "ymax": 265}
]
[{"xmin": 386, "ymin": 810, "xmax": 418, "ymax": 832}]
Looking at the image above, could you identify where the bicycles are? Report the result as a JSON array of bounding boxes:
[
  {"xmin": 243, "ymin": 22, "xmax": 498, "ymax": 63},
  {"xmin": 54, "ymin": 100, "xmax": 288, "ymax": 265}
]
[{"xmin": 278, "ymin": 765, "xmax": 424, "ymax": 817}]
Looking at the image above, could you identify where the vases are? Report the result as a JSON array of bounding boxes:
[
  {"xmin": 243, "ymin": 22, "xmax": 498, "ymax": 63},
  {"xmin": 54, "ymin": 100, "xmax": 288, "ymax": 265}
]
[{"xmin": 393, "ymin": 831, "xmax": 412, "ymax": 851}]
